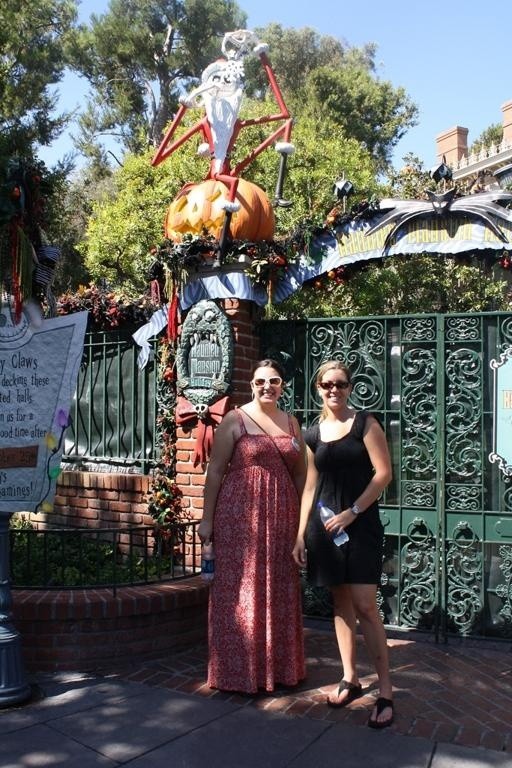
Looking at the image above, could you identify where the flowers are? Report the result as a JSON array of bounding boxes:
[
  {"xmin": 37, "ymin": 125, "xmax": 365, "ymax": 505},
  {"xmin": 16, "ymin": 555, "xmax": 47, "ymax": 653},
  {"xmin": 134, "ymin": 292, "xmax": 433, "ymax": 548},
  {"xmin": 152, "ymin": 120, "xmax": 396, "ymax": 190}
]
[
  {"xmin": 42, "ymin": 280, "xmax": 163, "ymax": 331},
  {"xmin": 147, "ymin": 332, "xmax": 184, "ymax": 545},
  {"xmin": 246, "ymin": 200, "xmax": 357, "ymax": 292}
]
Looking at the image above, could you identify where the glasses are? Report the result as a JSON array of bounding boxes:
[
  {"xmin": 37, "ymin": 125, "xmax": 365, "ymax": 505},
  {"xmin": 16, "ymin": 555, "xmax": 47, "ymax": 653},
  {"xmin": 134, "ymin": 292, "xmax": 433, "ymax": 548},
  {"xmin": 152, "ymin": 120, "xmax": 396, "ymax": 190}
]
[
  {"xmin": 252, "ymin": 376, "xmax": 282, "ymax": 387},
  {"xmin": 318, "ymin": 382, "xmax": 349, "ymax": 388}
]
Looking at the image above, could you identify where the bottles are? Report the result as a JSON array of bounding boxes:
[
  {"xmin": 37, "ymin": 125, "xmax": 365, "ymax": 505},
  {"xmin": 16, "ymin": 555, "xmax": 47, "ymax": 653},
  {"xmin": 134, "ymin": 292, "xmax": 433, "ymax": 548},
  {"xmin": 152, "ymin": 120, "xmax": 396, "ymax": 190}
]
[
  {"xmin": 314, "ymin": 501, "xmax": 350, "ymax": 546},
  {"xmin": 200, "ymin": 540, "xmax": 214, "ymax": 583}
]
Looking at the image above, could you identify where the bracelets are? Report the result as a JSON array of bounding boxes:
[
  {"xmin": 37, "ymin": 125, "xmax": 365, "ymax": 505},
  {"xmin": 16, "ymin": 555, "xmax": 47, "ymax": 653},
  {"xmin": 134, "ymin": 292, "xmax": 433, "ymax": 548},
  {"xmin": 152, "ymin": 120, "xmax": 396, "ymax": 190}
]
[{"xmin": 350, "ymin": 502, "xmax": 362, "ymax": 518}]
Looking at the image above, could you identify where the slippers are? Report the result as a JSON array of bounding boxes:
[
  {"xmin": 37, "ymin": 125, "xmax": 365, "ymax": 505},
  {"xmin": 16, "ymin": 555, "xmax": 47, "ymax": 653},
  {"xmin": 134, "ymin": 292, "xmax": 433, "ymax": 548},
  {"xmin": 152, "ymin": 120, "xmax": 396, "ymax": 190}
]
[
  {"xmin": 327, "ymin": 680, "xmax": 362, "ymax": 707},
  {"xmin": 368, "ymin": 697, "xmax": 393, "ymax": 728}
]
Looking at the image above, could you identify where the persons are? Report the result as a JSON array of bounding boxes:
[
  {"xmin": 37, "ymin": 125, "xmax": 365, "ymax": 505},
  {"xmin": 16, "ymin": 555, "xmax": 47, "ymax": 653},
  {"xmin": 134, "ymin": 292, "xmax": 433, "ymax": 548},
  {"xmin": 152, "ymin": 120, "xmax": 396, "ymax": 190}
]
[
  {"xmin": 288, "ymin": 359, "xmax": 394, "ymax": 731},
  {"xmin": 201, "ymin": 357, "xmax": 309, "ymax": 697}
]
